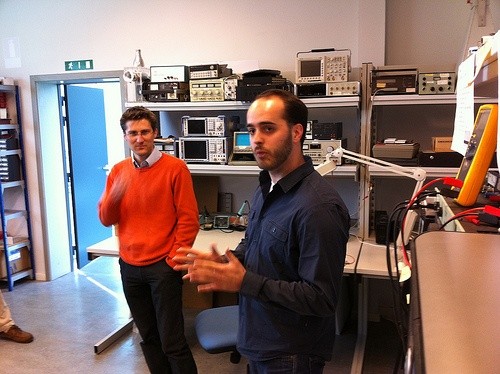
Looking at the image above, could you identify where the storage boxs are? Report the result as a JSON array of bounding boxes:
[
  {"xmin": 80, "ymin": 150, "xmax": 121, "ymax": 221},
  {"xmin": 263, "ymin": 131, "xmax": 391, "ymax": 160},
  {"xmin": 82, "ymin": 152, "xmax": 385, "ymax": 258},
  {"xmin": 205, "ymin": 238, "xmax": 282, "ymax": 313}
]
[
  {"xmin": 0, "ymin": 246, "xmax": 28, "ymax": 279},
  {"xmin": 0, "ymin": 78, "xmax": 14, "ymax": 85},
  {"xmin": 372, "ymin": 143, "xmax": 420, "ymax": 158},
  {"xmin": 432, "ymin": 136, "xmax": 455, "ymax": 152}
]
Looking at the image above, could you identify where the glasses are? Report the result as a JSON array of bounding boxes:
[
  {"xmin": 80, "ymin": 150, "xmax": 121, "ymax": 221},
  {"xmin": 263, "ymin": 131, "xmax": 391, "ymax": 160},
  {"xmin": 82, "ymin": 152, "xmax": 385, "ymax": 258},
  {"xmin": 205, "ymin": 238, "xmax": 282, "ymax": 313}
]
[{"xmin": 125, "ymin": 128, "xmax": 156, "ymax": 138}]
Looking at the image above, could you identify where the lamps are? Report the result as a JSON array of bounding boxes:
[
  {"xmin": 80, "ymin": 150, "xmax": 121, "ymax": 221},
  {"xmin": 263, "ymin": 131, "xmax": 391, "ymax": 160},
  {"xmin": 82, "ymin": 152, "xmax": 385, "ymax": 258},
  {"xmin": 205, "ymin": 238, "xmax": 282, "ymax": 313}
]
[
  {"xmin": 315, "ymin": 146, "xmax": 426, "ymax": 262},
  {"xmin": 236, "ymin": 200, "xmax": 250, "ymax": 218}
]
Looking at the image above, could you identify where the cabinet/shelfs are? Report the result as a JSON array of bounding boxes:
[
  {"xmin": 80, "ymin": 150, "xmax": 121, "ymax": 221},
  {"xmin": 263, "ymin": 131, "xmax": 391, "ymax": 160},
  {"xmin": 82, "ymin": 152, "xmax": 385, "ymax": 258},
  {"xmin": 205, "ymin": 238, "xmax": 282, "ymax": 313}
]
[
  {"xmin": 0, "ymin": 84, "xmax": 36, "ymax": 292},
  {"xmin": 87, "ymin": 63, "xmax": 500, "ymax": 374}
]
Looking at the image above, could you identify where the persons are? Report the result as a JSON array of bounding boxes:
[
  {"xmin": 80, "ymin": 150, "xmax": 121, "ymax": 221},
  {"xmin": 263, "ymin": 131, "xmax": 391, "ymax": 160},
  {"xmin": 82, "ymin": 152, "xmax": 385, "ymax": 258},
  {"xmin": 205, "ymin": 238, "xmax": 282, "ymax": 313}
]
[
  {"xmin": 97, "ymin": 106, "xmax": 200, "ymax": 374},
  {"xmin": 0, "ymin": 288, "xmax": 34, "ymax": 344},
  {"xmin": 170, "ymin": 88, "xmax": 352, "ymax": 374}
]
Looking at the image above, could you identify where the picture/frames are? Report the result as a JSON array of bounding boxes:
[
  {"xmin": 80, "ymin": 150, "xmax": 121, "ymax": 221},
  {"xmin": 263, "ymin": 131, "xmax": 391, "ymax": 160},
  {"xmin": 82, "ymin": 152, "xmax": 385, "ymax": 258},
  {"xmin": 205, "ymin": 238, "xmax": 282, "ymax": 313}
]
[{"xmin": 213, "ymin": 216, "xmax": 229, "ymax": 228}]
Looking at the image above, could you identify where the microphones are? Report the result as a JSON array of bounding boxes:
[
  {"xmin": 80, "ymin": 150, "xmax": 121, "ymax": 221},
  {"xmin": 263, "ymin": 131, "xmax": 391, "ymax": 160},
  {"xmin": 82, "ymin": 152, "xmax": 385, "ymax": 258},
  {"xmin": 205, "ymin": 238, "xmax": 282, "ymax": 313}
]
[{"xmin": 234, "ymin": 202, "xmax": 246, "ymax": 224}]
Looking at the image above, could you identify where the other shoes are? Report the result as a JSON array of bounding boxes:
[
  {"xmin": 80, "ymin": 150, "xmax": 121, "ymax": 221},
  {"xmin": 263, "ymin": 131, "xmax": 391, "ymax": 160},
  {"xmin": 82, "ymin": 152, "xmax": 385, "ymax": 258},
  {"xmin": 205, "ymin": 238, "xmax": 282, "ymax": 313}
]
[{"xmin": 0, "ymin": 325, "xmax": 33, "ymax": 343}]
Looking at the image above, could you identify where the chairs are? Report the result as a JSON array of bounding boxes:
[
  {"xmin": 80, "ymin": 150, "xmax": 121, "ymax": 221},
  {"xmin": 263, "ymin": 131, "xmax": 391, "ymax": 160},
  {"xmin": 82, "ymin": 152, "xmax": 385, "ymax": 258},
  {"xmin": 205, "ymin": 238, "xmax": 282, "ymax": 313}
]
[{"xmin": 195, "ymin": 304, "xmax": 242, "ymax": 364}]
